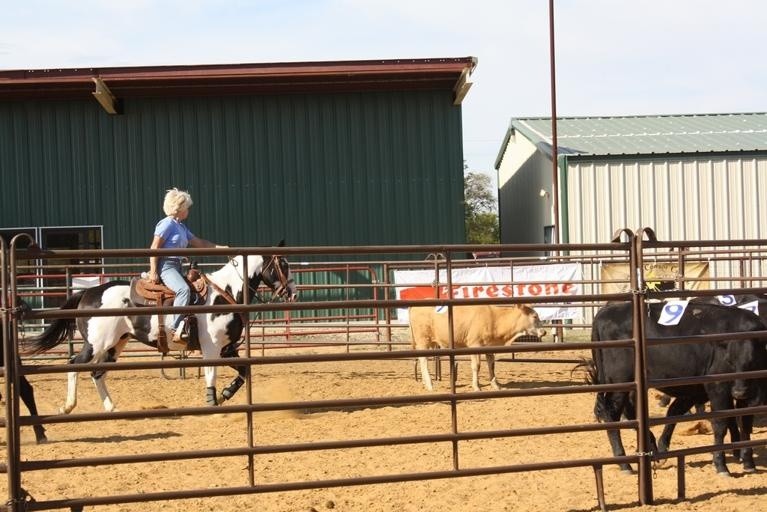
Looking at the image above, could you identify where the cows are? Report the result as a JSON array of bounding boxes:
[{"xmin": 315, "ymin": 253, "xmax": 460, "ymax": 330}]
[
  {"xmin": 570, "ymin": 293, "xmax": 767, "ymax": 478},
  {"xmin": 408, "ymin": 304, "xmax": 546, "ymax": 393}
]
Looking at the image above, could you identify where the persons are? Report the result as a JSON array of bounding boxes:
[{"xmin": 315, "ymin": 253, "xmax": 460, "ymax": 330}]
[{"xmin": 146, "ymin": 191, "xmax": 229, "ymax": 351}]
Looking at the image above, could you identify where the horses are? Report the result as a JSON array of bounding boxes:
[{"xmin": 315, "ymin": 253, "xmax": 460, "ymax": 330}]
[
  {"xmin": 0, "ymin": 293, "xmax": 49, "ymax": 445},
  {"xmin": 19, "ymin": 237, "xmax": 300, "ymax": 416}
]
